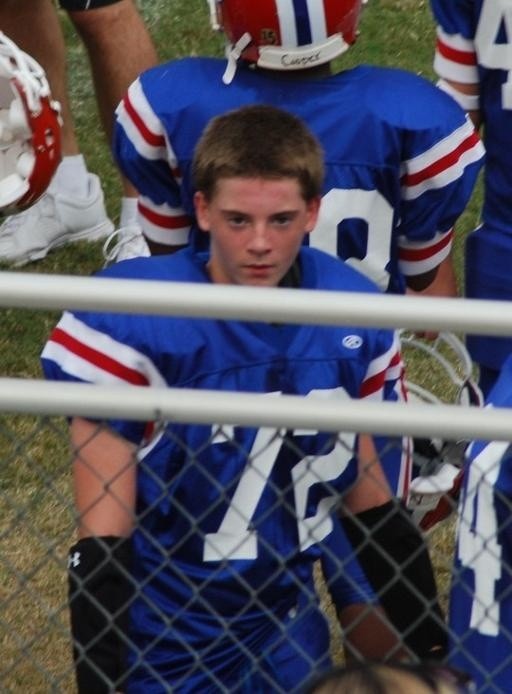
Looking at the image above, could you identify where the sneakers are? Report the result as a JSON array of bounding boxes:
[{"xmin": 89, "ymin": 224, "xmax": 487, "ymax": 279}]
[
  {"xmin": 115, "ymin": 225, "xmax": 152, "ymax": 263},
  {"xmin": 0, "ymin": 171, "xmax": 116, "ymax": 271}
]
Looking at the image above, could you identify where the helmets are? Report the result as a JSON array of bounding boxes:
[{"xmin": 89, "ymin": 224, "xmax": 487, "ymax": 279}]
[
  {"xmin": 400, "ymin": 383, "xmax": 485, "ymax": 531},
  {"xmin": 216, "ymin": 0, "xmax": 365, "ymax": 71}
]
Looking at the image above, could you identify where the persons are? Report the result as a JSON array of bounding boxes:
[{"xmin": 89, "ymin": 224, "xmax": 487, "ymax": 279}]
[
  {"xmin": 0, "ymin": 0, "xmax": 169, "ymax": 265},
  {"xmin": 448, "ymin": 363, "xmax": 512, "ymax": 692},
  {"xmin": 108, "ymin": 1, "xmax": 485, "ymax": 343},
  {"xmin": 421, "ymin": 0, "xmax": 512, "ymax": 401},
  {"xmin": 36, "ymin": 99, "xmax": 444, "ymax": 692}
]
